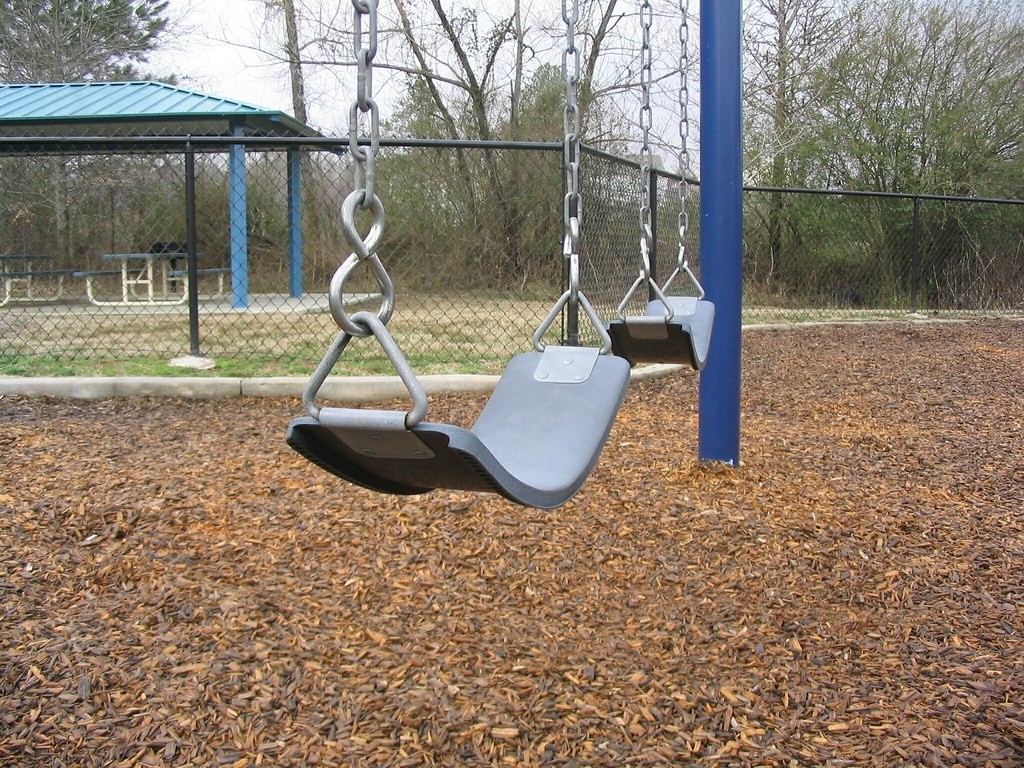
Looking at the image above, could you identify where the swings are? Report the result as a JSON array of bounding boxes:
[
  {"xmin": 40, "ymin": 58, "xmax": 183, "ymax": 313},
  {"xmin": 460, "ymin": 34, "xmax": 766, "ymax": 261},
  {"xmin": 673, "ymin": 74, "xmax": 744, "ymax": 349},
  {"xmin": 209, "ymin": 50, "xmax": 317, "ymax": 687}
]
[
  {"xmin": 602, "ymin": 1, "xmax": 716, "ymax": 373},
  {"xmin": 284, "ymin": 1, "xmax": 628, "ymax": 510}
]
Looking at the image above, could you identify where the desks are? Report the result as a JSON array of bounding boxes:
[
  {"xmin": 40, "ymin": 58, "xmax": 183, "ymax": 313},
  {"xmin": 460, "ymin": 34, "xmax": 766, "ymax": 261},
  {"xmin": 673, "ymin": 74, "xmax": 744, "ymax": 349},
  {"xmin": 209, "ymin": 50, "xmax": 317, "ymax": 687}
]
[
  {"xmin": 0, "ymin": 253, "xmax": 54, "ymax": 298},
  {"xmin": 98, "ymin": 252, "xmax": 207, "ymax": 301}
]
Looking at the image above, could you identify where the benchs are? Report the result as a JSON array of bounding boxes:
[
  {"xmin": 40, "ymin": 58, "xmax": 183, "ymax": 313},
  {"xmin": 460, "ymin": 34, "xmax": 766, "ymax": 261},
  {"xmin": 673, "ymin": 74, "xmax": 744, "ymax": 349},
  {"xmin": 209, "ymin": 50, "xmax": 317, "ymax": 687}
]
[{"xmin": 0, "ymin": 268, "xmax": 232, "ymax": 278}]
